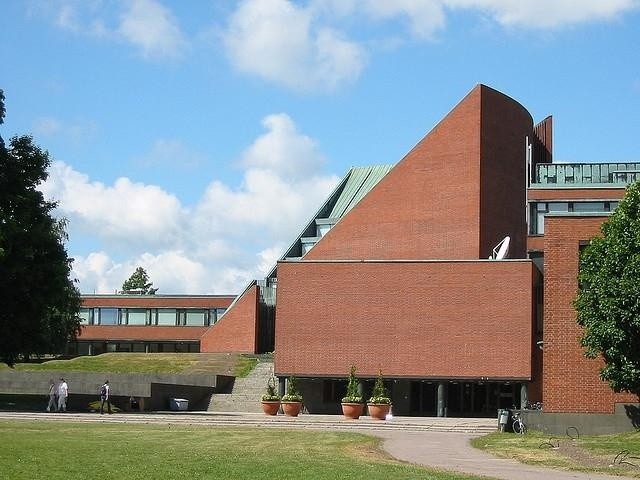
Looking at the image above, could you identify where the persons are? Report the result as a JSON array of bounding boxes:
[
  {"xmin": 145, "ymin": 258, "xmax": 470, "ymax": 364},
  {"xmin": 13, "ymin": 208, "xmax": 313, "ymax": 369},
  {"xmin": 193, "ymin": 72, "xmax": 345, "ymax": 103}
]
[
  {"xmin": 100, "ymin": 380, "xmax": 113, "ymax": 414},
  {"xmin": 55, "ymin": 377, "xmax": 68, "ymax": 412},
  {"xmin": 46, "ymin": 379, "xmax": 58, "ymax": 412}
]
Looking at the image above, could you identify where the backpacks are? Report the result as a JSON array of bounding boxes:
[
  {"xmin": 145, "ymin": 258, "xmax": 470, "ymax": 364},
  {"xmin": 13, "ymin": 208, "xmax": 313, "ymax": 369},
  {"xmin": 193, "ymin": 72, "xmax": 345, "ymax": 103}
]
[{"xmin": 99, "ymin": 385, "xmax": 109, "ymax": 396}]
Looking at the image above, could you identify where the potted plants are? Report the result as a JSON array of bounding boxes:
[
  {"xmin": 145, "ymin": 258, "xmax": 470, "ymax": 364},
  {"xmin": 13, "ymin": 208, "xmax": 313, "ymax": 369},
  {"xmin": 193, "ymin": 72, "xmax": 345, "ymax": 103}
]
[
  {"xmin": 340, "ymin": 363, "xmax": 392, "ymax": 419},
  {"xmin": 260, "ymin": 375, "xmax": 304, "ymax": 417}
]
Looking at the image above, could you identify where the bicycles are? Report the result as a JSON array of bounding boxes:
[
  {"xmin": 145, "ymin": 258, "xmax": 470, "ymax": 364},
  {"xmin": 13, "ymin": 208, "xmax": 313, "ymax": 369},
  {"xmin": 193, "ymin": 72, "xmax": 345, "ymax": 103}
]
[{"xmin": 512, "ymin": 413, "xmax": 527, "ymax": 434}]
